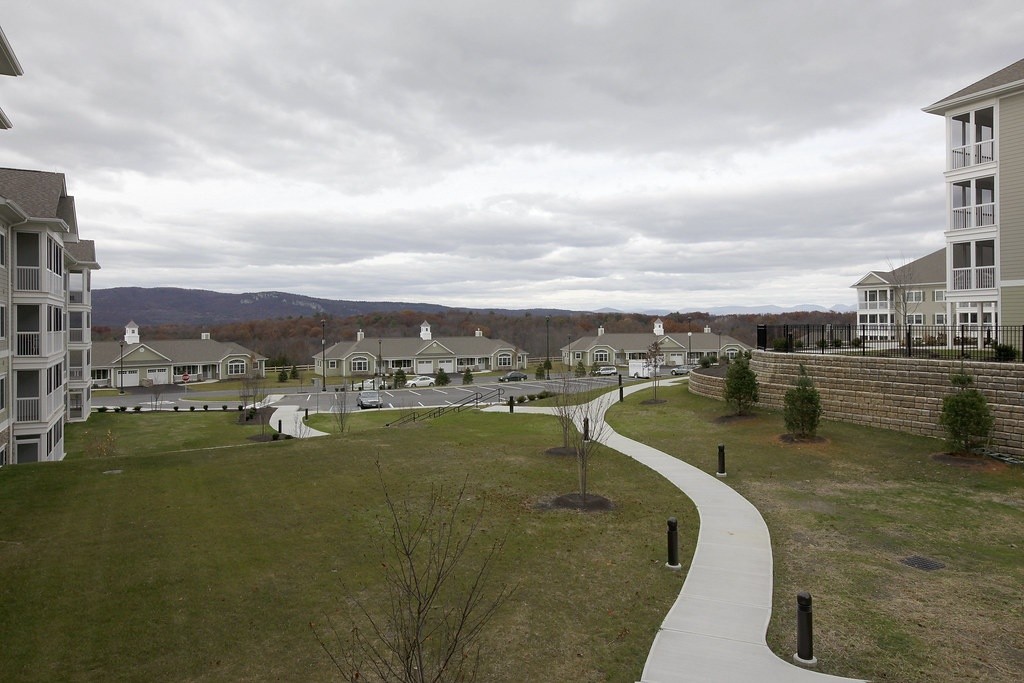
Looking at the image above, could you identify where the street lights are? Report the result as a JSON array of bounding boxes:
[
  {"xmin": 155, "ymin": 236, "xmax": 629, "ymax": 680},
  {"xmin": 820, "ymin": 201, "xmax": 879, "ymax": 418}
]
[
  {"xmin": 717, "ymin": 329, "xmax": 722, "ymax": 349},
  {"xmin": 321, "ymin": 317, "xmax": 327, "ymax": 392},
  {"xmin": 567, "ymin": 331, "xmax": 571, "ymax": 371},
  {"xmin": 545, "ymin": 312, "xmax": 552, "ymax": 380},
  {"xmin": 119, "ymin": 339, "xmax": 126, "ymax": 396},
  {"xmin": 377, "ymin": 334, "xmax": 384, "ymax": 389},
  {"xmin": 686, "ymin": 316, "xmax": 692, "ymax": 365}
]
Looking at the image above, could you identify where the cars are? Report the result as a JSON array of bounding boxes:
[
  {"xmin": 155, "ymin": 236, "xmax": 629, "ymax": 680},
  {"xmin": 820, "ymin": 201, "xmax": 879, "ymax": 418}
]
[
  {"xmin": 498, "ymin": 371, "xmax": 528, "ymax": 382},
  {"xmin": 404, "ymin": 376, "xmax": 436, "ymax": 388},
  {"xmin": 356, "ymin": 391, "xmax": 384, "ymax": 410}
]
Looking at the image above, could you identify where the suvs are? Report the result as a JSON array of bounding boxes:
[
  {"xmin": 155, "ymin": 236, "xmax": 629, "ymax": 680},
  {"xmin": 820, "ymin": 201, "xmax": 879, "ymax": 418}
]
[{"xmin": 670, "ymin": 365, "xmax": 695, "ymax": 376}]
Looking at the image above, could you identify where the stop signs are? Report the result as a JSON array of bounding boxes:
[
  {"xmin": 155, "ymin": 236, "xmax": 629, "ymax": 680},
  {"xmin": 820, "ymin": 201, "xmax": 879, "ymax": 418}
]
[{"xmin": 182, "ymin": 374, "xmax": 190, "ymax": 382}]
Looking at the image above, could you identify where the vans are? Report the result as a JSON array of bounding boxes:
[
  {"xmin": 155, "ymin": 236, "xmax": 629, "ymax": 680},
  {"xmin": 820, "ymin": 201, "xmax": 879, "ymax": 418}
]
[{"xmin": 595, "ymin": 366, "xmax": 617, "ymax": 376}]
